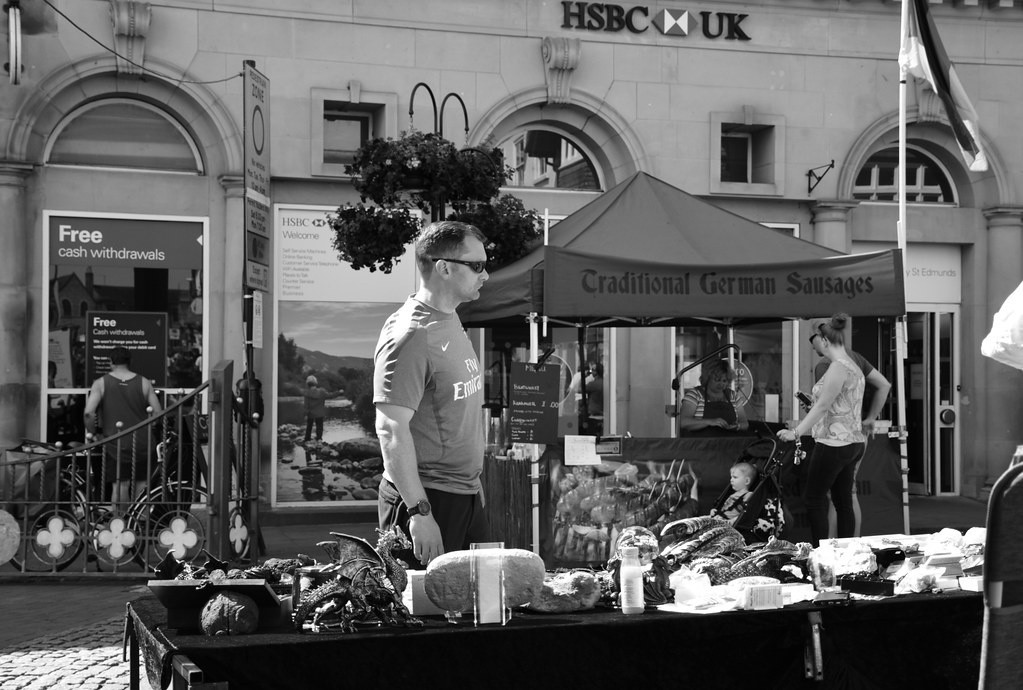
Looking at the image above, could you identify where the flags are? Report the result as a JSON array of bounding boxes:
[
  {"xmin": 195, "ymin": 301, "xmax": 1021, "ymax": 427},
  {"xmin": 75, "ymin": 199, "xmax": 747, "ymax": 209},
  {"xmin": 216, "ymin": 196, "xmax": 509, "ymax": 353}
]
[{"xmin": 899, "ymin": 0, "xmax": 989, "ymax": 171}]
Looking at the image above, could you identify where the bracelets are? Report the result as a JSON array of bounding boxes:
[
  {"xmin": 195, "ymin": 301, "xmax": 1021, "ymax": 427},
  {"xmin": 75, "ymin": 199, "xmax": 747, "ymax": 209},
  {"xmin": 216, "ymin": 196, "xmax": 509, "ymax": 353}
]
[
  {"xmin": 792, "ymin": 428, "xmax": 806, "ymax": 465},
  {"xmin": 736, "ymin": 423, "xmax": 740, "ymax": 431}
]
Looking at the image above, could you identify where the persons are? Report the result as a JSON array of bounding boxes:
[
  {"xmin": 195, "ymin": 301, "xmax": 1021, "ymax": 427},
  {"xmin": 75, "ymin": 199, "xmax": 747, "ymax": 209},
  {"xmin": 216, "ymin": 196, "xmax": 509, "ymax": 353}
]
[
  {"xmin": 777, "ymin": 313, "xmax": 891, "ymax": 549},
  {"xmin": 568, "ymin": 362, "xmax": 603, "ymax": 435},
  {"xmin": 680, "ymin": 359, "xmax": 748, "ymax": 431},
  {"xmin": 48, "ymin": 360, "xmax": 57, "ymax": 420},
  {"xmin": 302, "ymin": 375, "xmax": 343, "ymax": 442},
  {"xmin": 166, "ymin": 347, "xmax": 203, "ymax": 388},
  {"xmin": 85, "ymin": 346, "xmax": 161, "ymax": 516},
  {"xmin": 981, "ymin": 280, "xmax": 1023, "ymax": 371},
  {"xmin": 373, "ymin": 221, "xmax": 489, "ymax": 570},
  {"xmin": 710, "ymin": 462, "xmax": 757, "ymax": 523}
]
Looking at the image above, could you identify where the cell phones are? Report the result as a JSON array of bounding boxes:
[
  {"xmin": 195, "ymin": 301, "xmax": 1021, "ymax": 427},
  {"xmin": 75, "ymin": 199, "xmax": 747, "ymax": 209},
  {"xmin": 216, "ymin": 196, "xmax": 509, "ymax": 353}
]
[{"xmin": 795, "ymin": 391, "xmax": 814, "ymax": 408}]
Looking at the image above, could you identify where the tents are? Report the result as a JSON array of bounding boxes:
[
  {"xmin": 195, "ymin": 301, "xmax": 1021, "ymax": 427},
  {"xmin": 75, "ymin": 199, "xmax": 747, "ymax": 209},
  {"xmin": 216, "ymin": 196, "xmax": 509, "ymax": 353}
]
[{"xmin": 457, "ymin": 172, "xmax": 905, "ymax": 571}]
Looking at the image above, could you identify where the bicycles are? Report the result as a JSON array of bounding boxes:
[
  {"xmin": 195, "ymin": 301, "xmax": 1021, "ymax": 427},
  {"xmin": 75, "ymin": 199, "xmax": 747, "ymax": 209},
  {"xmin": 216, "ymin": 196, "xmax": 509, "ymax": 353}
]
[{"xmin": 2, "ymin": 432, "xmax": 219, "ymax": 575}]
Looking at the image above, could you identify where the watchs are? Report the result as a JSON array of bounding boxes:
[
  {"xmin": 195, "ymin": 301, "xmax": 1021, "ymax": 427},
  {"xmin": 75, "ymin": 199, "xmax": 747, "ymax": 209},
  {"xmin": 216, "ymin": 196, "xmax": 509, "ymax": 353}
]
[{"xmin": 406, "ymin": 499, "xmax": 431, "ymax": 517}]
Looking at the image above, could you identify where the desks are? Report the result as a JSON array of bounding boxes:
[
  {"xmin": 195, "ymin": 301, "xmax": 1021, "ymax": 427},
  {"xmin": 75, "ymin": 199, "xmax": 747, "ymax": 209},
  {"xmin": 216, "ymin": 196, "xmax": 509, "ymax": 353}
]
[{"xmin": 121, "ymin": 584, "xmax": 985, "ymax": 690}]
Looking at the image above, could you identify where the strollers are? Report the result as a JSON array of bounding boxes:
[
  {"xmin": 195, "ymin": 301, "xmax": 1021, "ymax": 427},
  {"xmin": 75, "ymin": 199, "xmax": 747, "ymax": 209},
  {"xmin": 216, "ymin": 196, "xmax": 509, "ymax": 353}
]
[{"xmin": 672, "ymin": 438, "xmax": 794, "ymax": 544}]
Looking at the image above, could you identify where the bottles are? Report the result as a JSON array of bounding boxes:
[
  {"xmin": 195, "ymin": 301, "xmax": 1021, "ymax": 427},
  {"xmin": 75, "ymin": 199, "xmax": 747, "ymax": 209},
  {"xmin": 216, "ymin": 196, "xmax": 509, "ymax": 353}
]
[{"xmin": 620, "ymin": 546, "xmax": 645, "ymax": 614}]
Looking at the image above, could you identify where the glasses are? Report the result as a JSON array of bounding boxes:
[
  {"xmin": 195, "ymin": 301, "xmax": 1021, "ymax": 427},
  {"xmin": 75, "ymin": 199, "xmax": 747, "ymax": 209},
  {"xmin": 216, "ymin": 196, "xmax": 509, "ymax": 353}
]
[
  {"xmin": 431, "ymin": 257, "xmax": 486, "ymax": 275},
  {"xmin": 809, "ymin": 331, "xmax": 819, "ymax": 344}
]
